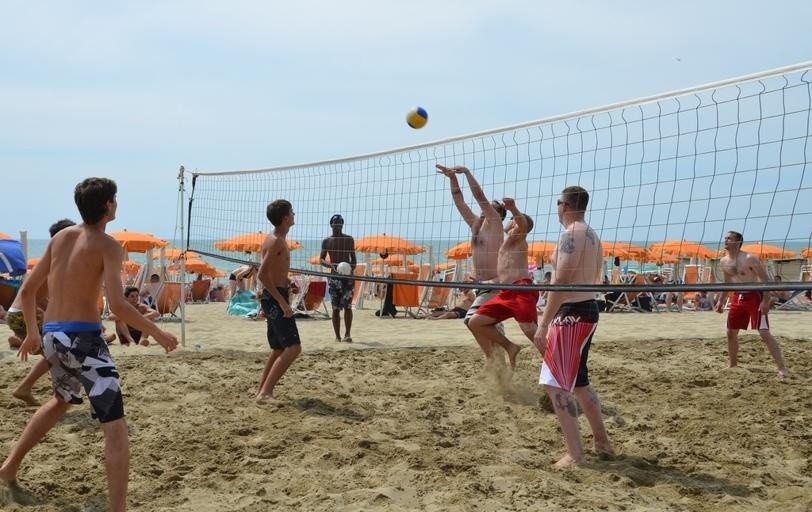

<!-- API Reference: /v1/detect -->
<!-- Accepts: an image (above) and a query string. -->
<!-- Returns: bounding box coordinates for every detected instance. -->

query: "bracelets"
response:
[449,188,460,195]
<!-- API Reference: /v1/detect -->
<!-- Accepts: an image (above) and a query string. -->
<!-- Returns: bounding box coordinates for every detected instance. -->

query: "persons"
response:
[255,199,302,405]
[603,262,723,312]
[713,231,789,376]
[101,327,116,343]
[434,164,507,371]
[536,272,551,300]
[0,176,180,510]
[468,198,547,370]
[534,186,616,468]
[8,332,23,348]
[108,287,160,345]
[6,219,79,406]
[320,213,357,341]
[770,275,812,309]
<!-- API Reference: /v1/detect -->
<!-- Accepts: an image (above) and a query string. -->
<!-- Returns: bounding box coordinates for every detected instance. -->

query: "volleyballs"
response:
[337,263,352,276]
[407,108,428,129]
